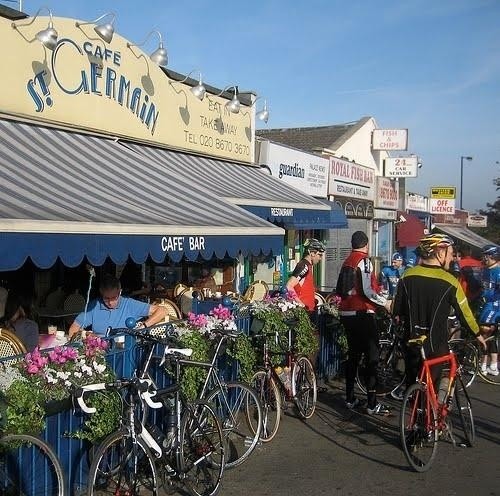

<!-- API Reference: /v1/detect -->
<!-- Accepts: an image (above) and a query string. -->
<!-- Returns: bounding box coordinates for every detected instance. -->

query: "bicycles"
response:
[244,318,317,442]
[400,325,476,473]
[77,349,225,496]
[0,434,66,496]
[473,322,500,386]
[354,319,479,397]
[87,326,263,478]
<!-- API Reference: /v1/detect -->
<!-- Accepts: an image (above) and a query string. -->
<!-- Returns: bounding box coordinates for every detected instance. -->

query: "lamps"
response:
[12,5,270,124]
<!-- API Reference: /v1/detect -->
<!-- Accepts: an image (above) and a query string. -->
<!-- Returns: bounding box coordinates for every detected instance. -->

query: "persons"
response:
[335,231,393,418]
[1,268,174,361]
[377,245,500,401]
[387,233,490,455]
[285,236,329,394]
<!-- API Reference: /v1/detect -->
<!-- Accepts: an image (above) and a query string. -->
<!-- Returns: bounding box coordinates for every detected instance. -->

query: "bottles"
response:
[274,362,288,383]
[437,374,450,405]
[165,407,177,437]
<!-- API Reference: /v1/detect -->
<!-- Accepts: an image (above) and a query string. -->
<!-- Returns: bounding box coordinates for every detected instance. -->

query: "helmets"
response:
[481,244,500,260]
[303,238,326,253]
[420,233,455,258]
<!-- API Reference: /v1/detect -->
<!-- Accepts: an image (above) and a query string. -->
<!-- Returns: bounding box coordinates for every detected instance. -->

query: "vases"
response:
[35,394,87,418]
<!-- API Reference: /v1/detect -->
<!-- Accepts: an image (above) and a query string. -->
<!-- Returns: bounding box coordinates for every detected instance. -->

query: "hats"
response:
[351,231,369,248]
[392,252,403,260]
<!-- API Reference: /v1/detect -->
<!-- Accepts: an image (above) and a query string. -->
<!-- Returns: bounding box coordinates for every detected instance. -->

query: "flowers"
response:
[166,291,320,400]
[326,295,348,354]
[0,335,121,448]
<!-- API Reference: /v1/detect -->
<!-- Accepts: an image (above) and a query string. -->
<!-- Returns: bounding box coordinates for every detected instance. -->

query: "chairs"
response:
[0,265,269,364]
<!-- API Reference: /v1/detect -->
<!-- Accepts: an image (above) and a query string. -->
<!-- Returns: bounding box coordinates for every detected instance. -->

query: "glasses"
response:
[103,290,120,301]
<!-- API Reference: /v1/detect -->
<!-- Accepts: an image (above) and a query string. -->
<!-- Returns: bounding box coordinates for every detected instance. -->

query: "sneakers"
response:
[468,367,488,375]
[487,367,499,375]
[344,398,368,408]
[367,402,392,416]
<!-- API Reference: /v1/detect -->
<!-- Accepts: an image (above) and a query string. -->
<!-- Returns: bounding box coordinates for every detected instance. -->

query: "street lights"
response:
[460,156,473,210]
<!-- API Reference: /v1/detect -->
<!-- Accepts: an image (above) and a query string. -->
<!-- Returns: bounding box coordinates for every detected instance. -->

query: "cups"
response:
[47,325,65,338]
